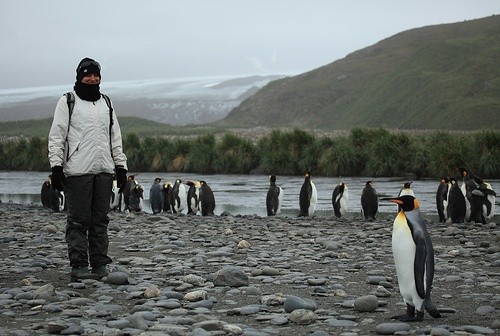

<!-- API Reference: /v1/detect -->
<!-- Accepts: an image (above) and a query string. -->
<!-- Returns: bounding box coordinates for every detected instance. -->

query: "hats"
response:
[76,58,101,83]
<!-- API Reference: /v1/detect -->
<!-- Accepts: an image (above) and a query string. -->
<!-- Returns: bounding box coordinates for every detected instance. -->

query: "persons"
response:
[47,58,127,280]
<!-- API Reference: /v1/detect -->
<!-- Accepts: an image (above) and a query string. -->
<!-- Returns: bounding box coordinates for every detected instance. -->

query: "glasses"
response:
[78,61,101,75]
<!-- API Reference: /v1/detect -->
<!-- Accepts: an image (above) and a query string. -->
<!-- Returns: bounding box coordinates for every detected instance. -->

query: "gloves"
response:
[51,166,68,191]
[115,165,128,194]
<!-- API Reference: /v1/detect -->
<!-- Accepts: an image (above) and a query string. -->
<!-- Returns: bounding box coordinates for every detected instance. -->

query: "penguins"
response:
[39,166,500,226]
[377,193,444,323]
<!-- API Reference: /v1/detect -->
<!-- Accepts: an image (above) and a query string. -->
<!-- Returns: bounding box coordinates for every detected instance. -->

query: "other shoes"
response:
[71,267,99,279]
[91,265,109,280]
